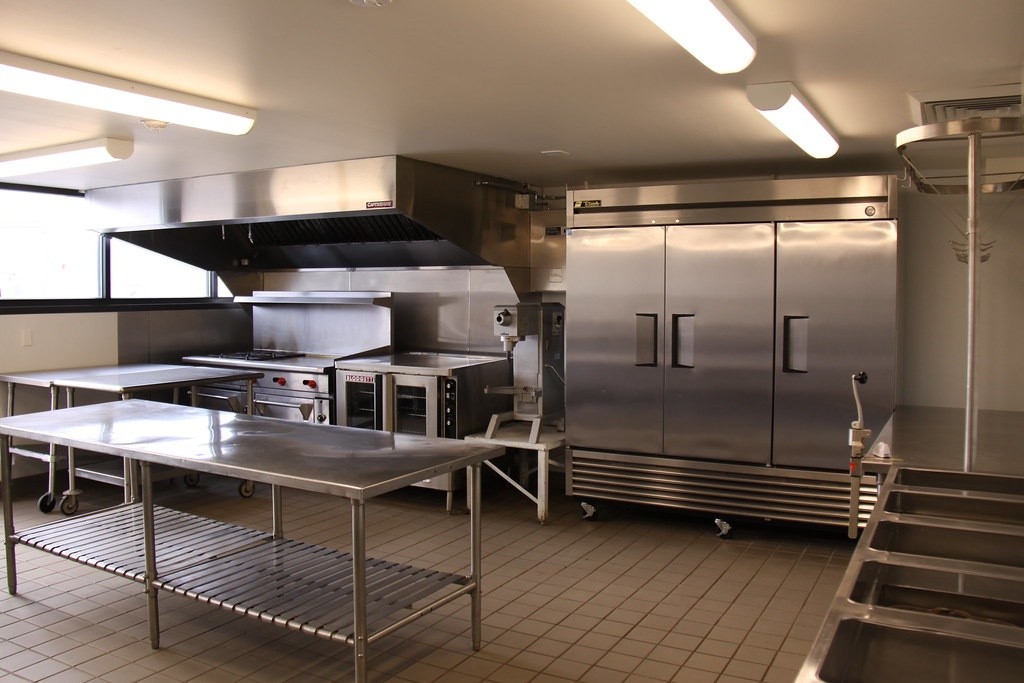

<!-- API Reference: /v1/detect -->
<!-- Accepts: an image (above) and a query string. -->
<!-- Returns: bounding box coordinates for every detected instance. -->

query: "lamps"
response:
[745,81,840,158]
[0,137,134,175]
[0,50,257,135]
[624,0,756,74]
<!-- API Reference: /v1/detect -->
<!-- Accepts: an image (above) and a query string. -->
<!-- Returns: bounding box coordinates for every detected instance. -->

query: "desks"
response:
[52,366,264,514]
[0,362,193,512]
[0,398,506,683]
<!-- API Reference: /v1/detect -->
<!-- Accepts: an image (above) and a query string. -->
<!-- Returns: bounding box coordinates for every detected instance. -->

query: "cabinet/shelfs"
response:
[563,175,907,539]
